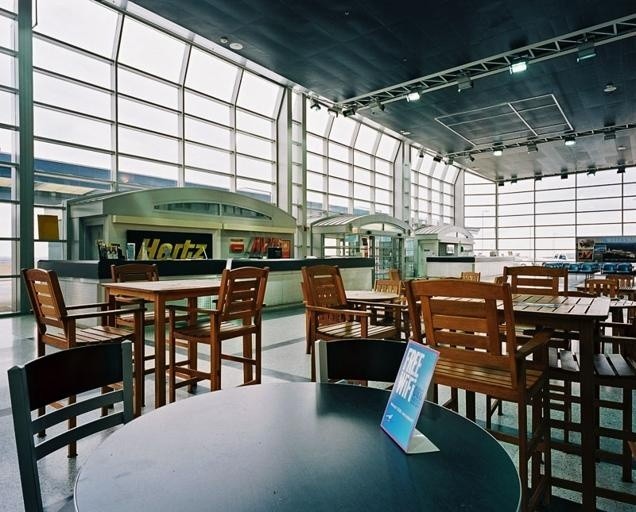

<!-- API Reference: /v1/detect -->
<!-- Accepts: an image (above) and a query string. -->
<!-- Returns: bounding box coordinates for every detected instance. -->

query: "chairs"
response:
[314,336,414,384]
[503,264,572,453]
[538,346,636,511]
[404,278,552,512]
[167,265,270,402]
[110,262,182,406]
[298,264,408,387]
[6,338,136,510]
[422,255,636,360]
[19,267,145,458]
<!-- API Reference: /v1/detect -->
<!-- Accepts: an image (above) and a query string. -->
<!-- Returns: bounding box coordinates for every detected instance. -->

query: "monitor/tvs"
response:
[267,247,283,259]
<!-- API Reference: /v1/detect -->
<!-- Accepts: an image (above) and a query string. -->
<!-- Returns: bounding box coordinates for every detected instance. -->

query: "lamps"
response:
[308,96,343,118]
[340,37,597,118]
[442,126,619,166]
[496,160,629,187]
[416,146,444,163]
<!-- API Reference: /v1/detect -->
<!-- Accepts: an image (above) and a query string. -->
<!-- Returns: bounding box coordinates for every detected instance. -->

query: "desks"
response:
[73,382,522,511]
[403,293,611,509]
[96,279,265,429]
